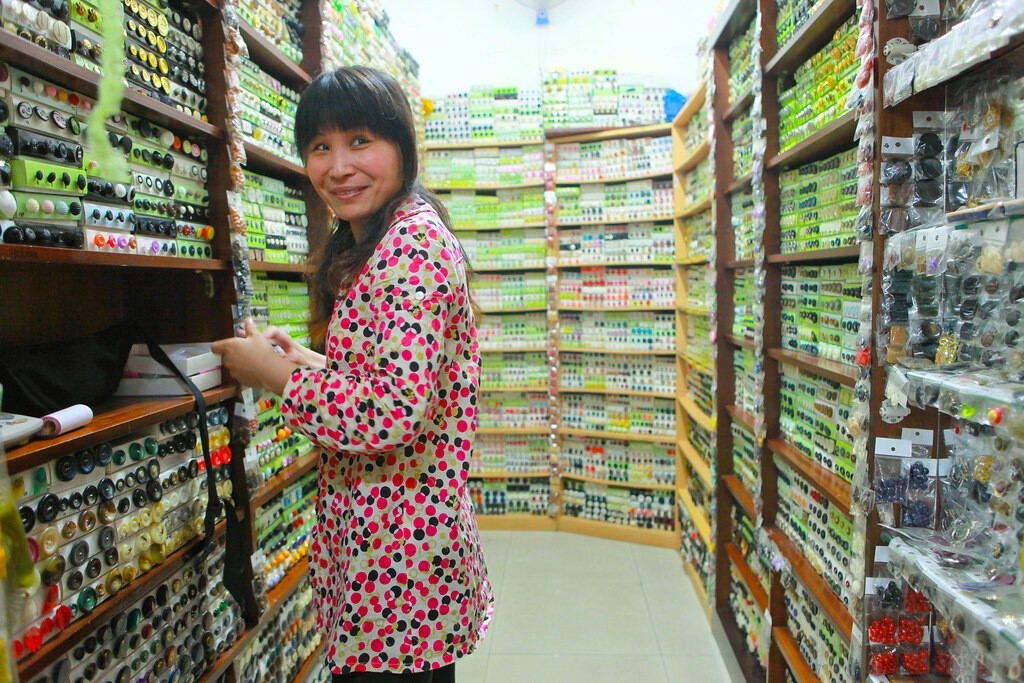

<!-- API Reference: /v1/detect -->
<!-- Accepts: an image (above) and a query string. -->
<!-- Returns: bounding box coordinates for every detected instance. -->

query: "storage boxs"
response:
[0,0,862,683]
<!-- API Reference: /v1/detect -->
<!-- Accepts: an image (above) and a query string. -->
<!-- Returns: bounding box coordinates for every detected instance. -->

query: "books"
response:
[30,404,93,437]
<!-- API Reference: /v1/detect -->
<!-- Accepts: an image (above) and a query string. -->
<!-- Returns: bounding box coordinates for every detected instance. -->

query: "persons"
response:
[211,65,495,683]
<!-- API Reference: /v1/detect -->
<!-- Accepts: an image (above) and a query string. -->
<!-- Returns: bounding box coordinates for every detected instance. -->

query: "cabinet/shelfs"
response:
[2,0,420,683]
[425,0,1024,683]
